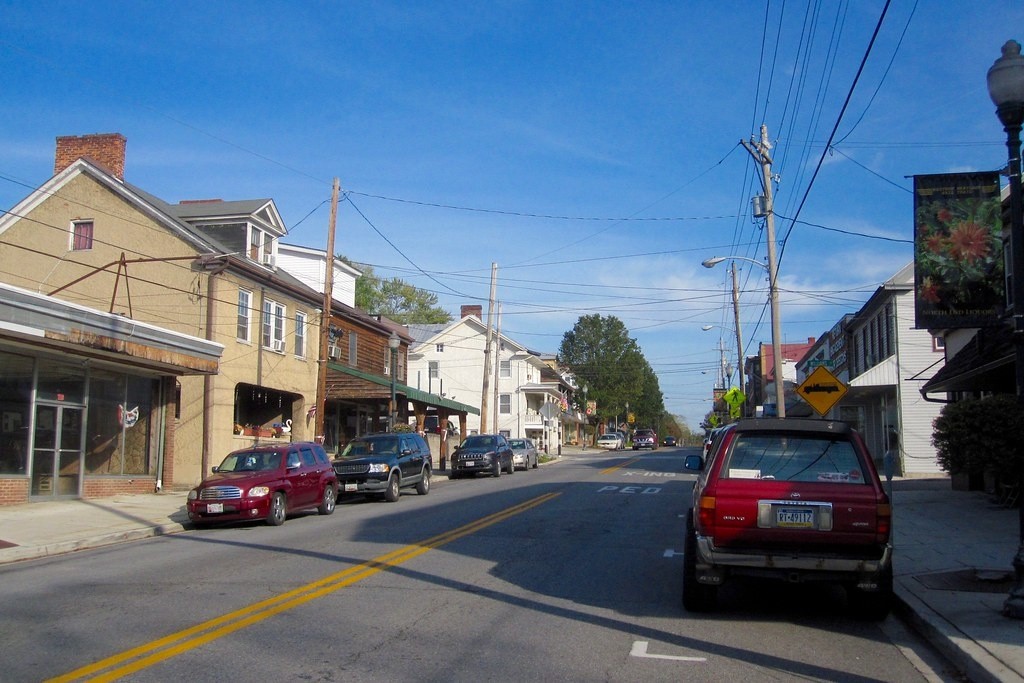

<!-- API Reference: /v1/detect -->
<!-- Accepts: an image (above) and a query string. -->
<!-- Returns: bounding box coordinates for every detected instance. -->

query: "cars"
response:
[606,432,626,450]
[502,437,539,471]
[702,423,746,467]
[597,433,622,451]
[663,435,676,447]
[683,416,896,615]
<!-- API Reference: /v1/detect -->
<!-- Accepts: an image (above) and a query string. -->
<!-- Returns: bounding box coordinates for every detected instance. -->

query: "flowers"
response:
[233,421,285,439]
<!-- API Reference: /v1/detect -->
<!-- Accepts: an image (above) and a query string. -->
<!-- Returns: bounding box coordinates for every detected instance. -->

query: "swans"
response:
[281,419,292,433]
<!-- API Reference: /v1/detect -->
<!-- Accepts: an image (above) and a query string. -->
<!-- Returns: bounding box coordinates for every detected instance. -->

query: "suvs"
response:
[449,433,514,479]
[331,431,433,500]
[187,441,336,528]
[631,428,657,450]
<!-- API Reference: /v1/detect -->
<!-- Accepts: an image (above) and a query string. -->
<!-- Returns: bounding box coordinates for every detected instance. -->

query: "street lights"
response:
[700,123,792,422]
[700,364,725,425]
[387,330,401,430]
[581,384,588,452]
[624,401,630,426]
[702,261,749,418]
